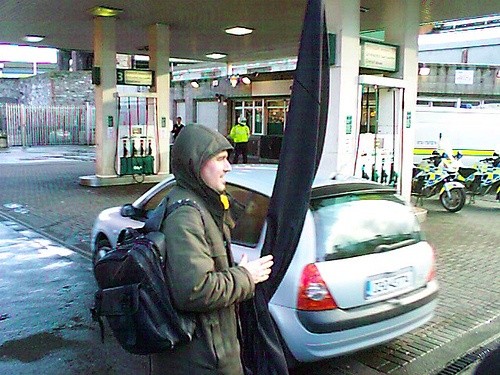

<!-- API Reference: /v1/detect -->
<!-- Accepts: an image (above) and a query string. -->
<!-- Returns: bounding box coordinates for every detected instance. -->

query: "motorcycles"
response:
[411,139,467,212]
[455,152,500,204]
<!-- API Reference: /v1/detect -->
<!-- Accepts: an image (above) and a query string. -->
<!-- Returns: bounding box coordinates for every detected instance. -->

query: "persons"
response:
[132,124,275,375]
[172,116,184,140]
[225,116,251,165]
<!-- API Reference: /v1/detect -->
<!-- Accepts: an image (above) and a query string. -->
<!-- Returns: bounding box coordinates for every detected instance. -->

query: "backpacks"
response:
[90,197,207,356]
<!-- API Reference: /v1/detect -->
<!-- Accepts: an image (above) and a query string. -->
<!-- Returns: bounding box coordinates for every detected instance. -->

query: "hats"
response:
[238,117,247,125]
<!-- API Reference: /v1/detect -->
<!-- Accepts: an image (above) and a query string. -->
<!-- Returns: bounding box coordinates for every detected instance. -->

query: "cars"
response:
[90,163,439,375]
